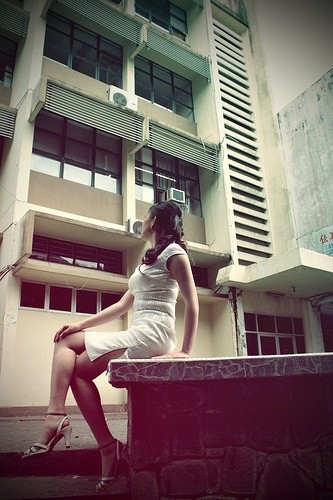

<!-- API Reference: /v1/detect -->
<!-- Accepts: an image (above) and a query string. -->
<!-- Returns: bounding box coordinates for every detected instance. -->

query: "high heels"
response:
[96,438,128,493]
[20,412,72,459]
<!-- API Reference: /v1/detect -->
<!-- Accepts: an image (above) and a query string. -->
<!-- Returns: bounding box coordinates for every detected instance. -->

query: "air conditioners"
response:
[165,187,185,204]
[125,218,143,234]
[105,85,138,111]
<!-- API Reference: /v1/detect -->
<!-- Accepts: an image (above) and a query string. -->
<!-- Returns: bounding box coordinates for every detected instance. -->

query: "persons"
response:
[21,200,200,492]
